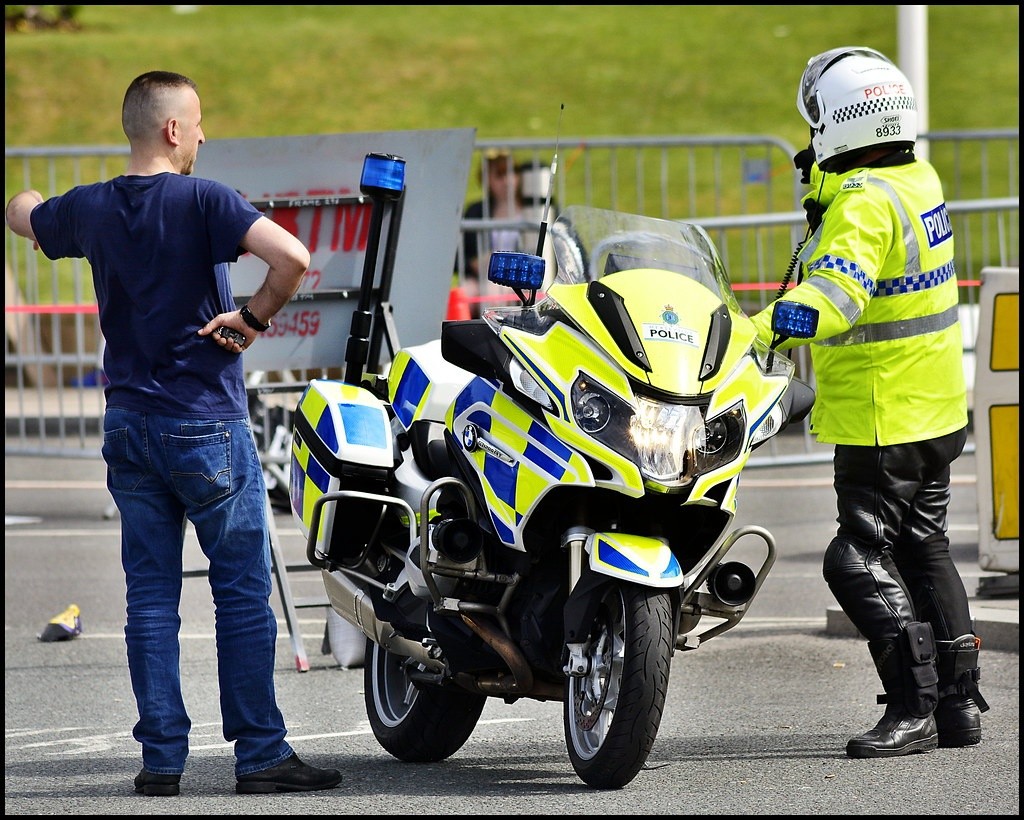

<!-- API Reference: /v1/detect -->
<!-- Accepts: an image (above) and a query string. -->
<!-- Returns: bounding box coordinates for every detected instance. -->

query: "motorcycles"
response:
[288,153,820,788]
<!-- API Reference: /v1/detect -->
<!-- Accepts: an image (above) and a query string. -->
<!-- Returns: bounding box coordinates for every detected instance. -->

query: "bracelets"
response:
[240,306,271,332]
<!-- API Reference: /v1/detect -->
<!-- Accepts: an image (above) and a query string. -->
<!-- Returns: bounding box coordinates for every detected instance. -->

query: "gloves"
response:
[794,149,816,185]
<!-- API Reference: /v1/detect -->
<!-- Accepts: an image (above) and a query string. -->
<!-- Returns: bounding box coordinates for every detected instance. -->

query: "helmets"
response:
[797,47,917,176]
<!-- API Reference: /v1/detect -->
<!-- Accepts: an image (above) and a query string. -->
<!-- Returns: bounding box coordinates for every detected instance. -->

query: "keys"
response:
[219,326,246,347]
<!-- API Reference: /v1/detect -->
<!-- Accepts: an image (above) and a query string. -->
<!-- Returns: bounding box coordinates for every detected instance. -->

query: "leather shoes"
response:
[134,767,181,797]
[933,698,981,748]
[235,751,344,795]
[846,705,938,758]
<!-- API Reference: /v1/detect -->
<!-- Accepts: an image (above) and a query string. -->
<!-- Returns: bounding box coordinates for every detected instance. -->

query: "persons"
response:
[7,70,343,795]
[747,45,989,758]
[465,148,525,277]
